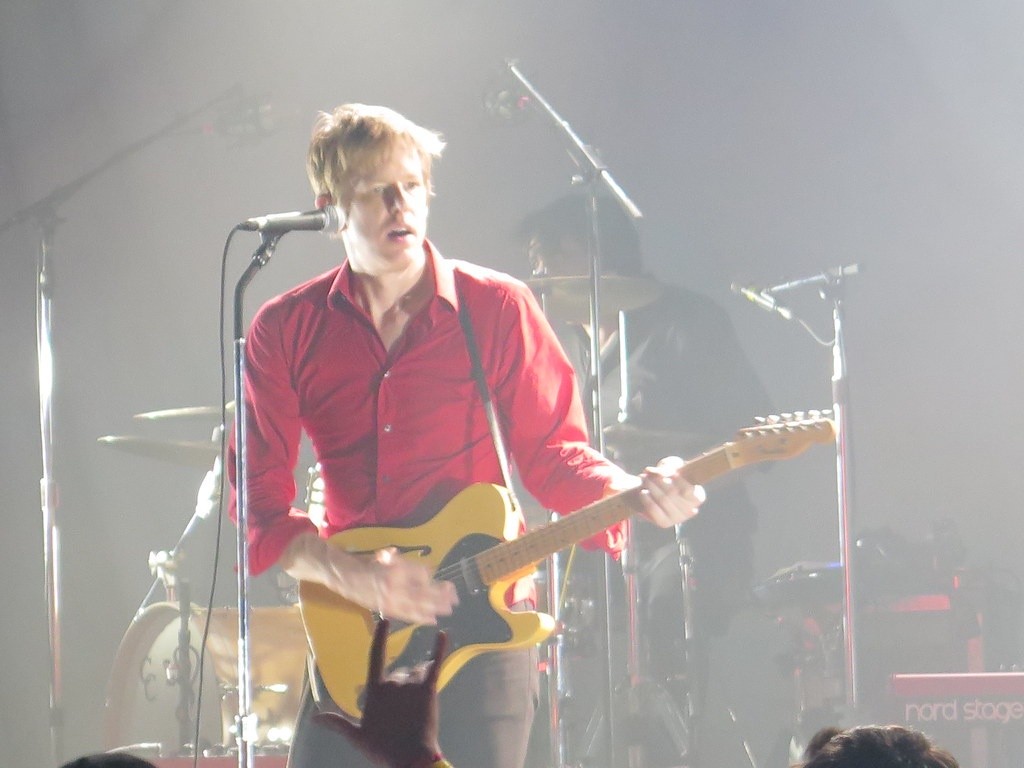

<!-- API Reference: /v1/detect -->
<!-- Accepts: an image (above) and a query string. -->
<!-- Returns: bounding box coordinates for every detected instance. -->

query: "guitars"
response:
[298,410,835,721]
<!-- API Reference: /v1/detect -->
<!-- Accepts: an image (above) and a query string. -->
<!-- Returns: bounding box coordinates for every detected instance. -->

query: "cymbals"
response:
[520,274,662,317]
[97,435,224,464]
[133,400,237,422]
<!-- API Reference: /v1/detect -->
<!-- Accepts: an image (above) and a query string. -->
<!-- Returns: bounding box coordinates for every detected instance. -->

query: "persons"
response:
[515,184,774,768]
[805,723,961,768]
[229,99,710,768]
[59,614,462,766]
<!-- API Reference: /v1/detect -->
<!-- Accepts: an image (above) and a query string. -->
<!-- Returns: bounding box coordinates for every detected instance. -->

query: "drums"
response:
[105,600,309,754]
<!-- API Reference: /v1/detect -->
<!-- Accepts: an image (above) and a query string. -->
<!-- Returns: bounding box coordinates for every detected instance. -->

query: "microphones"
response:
[481,86,538,123]
[197,401,238,520]
[238,204,347,234]
[731,281,791,321]
